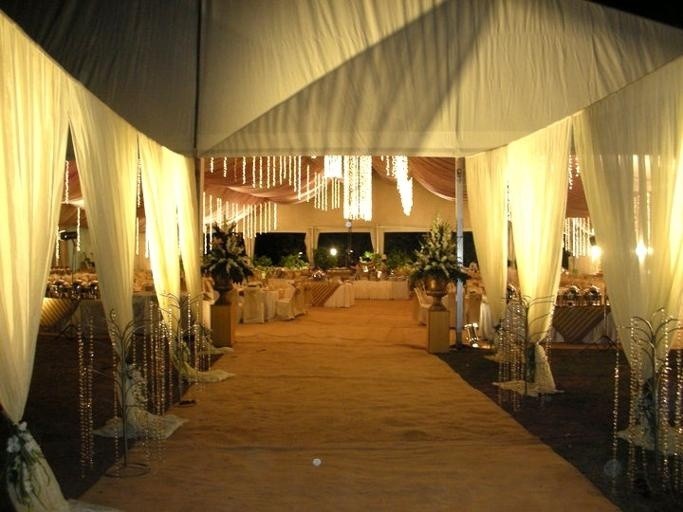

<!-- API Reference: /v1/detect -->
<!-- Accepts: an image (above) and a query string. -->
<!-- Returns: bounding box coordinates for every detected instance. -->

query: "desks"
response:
[302,280,355,308]
[238,287,294,320]
[345,277,411,300]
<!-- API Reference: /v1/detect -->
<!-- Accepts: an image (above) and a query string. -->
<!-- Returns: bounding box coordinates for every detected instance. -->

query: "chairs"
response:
[271,279,306,321]
[243,287,264,323]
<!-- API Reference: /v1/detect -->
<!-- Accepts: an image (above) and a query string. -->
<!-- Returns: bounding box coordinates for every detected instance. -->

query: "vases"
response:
[213,275,232,304]
[425,277,449,311]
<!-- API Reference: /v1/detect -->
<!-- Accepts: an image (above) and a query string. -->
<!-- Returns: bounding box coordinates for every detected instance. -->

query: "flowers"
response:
[201,223,254,283]
[411,217,471,284]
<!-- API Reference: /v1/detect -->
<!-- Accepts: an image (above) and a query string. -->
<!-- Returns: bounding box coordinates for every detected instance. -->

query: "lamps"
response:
[589,236,602,258]
